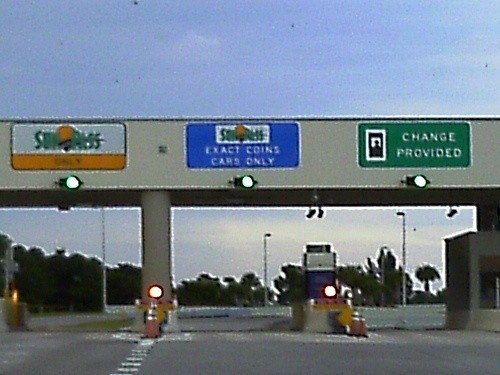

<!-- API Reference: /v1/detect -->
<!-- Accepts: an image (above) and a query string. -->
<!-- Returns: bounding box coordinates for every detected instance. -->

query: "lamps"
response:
[401,174,431,188]
[55,175,85,189]
[228,174,259,189]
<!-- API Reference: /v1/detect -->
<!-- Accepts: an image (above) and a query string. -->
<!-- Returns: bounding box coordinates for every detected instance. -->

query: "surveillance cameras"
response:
[318,210,324,218]
[307,210,316,218]
[447,209,457,217]
